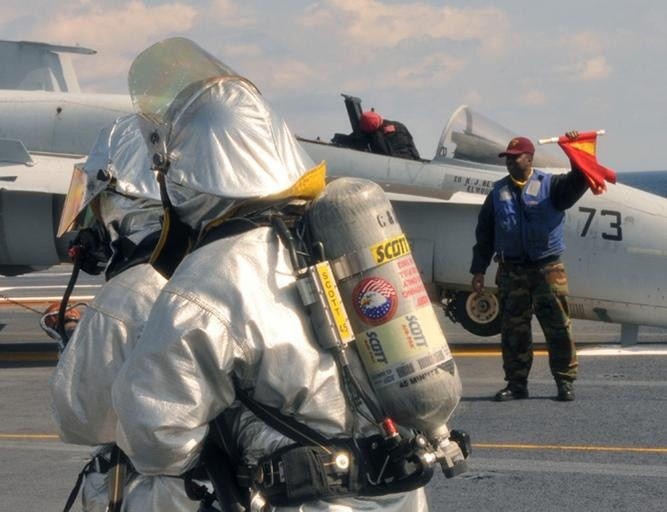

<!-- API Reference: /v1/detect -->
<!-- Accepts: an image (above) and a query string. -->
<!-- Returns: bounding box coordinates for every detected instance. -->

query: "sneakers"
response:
[557,381,576,401]
[495,383,529,401]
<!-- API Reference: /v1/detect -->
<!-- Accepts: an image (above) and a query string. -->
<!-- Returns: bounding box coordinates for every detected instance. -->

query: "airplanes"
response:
[0,41,667,338]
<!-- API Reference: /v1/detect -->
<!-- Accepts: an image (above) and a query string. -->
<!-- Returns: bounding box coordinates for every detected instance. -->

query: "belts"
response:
[497,254,561,271]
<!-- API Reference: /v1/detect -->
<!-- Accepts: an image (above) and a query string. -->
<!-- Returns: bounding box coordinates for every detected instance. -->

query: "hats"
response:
[498,136,535,157]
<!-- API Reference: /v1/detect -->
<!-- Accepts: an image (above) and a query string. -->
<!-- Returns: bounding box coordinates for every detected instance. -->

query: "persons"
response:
[108,35,431,510]
[47,113,220,510]
[467,130,591,402]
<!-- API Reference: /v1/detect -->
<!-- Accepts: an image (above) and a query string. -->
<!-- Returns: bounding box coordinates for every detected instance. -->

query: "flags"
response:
[556,131,616,196]
